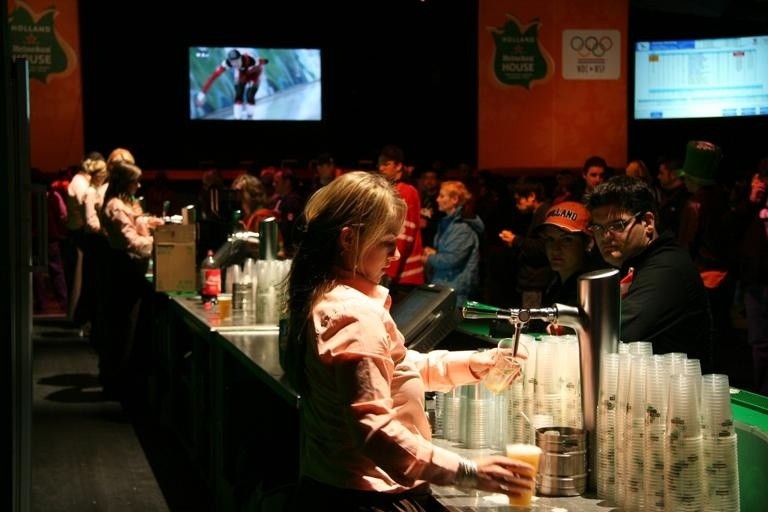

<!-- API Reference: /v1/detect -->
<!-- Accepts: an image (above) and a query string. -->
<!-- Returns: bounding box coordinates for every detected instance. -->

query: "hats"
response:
[540,201,593,235]
[228,50,241,61]
[674,139,724,186]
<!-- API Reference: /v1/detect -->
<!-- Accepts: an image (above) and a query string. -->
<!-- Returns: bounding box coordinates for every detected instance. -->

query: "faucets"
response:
[209,217,280,281]
[460,266,620,502]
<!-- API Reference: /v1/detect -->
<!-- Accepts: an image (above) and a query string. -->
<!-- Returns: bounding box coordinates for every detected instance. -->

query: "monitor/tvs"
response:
[630,35,768,121]
[186,44,326,126]
[390,281,458,349]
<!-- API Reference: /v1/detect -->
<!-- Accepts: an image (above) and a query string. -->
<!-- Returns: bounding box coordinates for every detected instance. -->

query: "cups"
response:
[216,294,234,320]
[507,444,542,507]
[233,282,253,311]
[435,348,507,450]
[498,333,583,449]
[255,258,295,322]
[596,339,743,512]
[224,257,254,293]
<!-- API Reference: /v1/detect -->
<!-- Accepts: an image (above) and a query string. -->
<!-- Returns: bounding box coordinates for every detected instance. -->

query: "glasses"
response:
[585,209,647,236]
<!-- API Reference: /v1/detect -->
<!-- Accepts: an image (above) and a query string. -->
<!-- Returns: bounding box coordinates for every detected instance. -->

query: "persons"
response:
[61,145,767,511]
[197,50,268,120]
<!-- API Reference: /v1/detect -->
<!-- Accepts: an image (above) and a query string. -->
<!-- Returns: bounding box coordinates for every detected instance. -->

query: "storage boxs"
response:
[152,224,196,292]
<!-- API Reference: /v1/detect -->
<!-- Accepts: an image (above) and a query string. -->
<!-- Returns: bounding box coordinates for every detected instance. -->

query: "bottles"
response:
[200,249,221,303]
